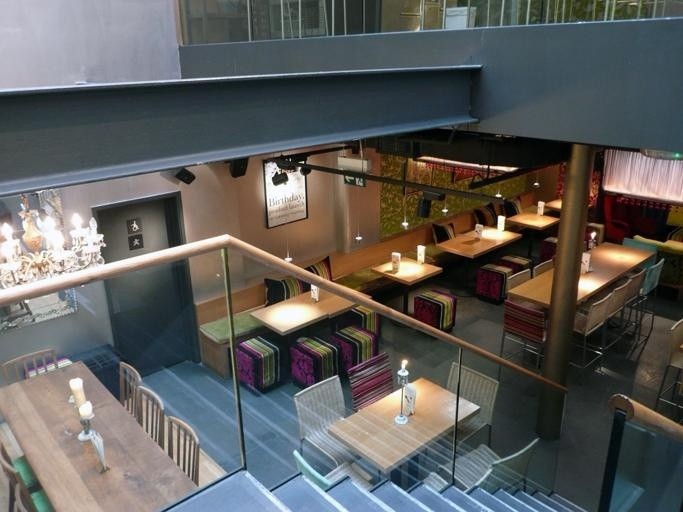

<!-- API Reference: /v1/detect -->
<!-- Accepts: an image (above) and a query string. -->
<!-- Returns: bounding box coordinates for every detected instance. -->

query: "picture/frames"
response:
[262,157,308,228]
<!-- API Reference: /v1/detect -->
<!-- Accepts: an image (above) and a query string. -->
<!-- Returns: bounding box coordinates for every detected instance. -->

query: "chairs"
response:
[505,269,531,289]
[569,292,612,387]
[0,348,200,512]
[438,438,541,494]
[497,300,549,380]
[655,319,683,411]
[417,362,499,479]
[533,259,552,278]
[294,375,361,468]
[347,351,394,413]
[602,258,665,342]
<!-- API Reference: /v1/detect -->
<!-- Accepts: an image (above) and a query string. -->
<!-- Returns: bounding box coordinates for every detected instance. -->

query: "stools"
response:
[414,290,457,331]
[329,325,379,378]
[236,335,283,393]
[502,255,533,273]
[475,262,515,303]
[292,336,340,387]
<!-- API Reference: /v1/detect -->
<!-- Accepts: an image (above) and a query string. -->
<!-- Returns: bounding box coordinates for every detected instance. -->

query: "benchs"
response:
[194,282,267,381]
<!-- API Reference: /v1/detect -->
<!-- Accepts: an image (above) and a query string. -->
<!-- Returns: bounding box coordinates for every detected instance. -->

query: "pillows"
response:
[501,197,522,218]
[265,255,333,307]
[432,223,455,245]
[472,200,496,228]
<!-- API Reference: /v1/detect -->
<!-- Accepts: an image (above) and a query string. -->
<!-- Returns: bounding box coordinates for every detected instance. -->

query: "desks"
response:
[546,197,591,213]
[505,210,561,258]
[435,225,522,285]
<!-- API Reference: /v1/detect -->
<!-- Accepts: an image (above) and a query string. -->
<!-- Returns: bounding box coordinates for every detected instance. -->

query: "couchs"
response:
[597,190,683,290]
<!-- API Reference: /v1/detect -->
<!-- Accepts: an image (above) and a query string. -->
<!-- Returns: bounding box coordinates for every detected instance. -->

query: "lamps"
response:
[0,193,105,291]
[175,127,469,220]
